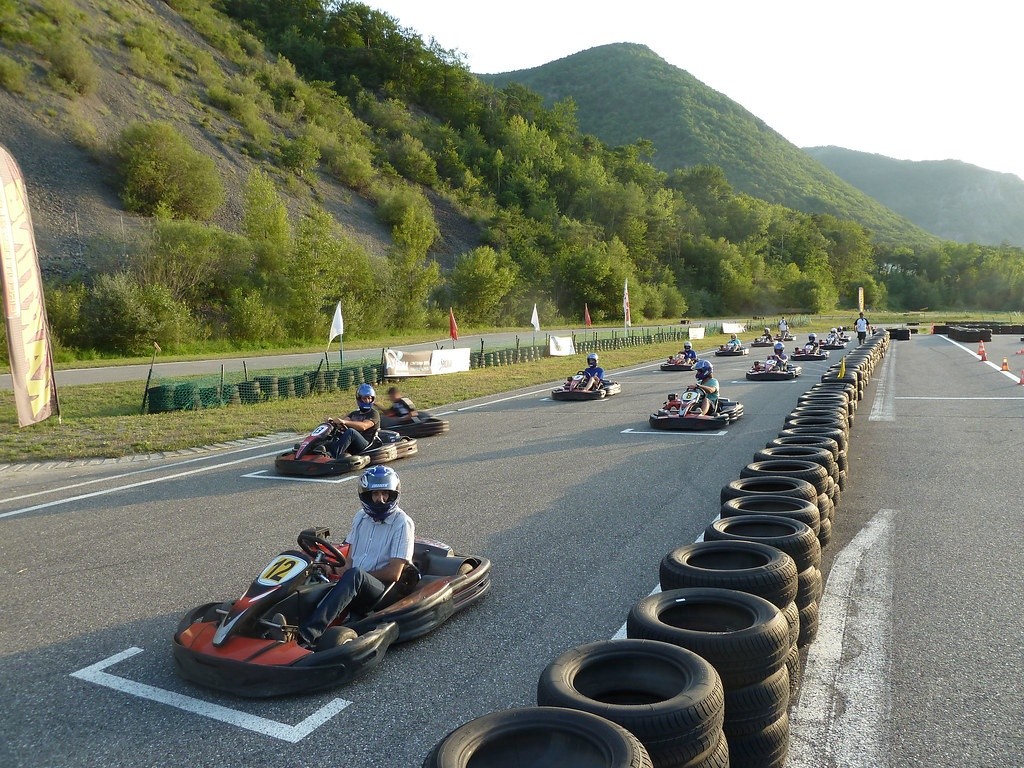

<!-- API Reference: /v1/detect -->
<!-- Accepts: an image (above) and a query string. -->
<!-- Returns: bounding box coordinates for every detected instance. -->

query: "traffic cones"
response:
[977,340,984,355]
[980,350,989,361]
[1000,356,1010,371]
[1017,370,1024,385]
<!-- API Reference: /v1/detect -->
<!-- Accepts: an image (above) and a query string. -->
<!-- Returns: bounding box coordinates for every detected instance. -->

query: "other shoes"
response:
[312,449,329,457]
[696,408,703,415]
[269,612,295,644]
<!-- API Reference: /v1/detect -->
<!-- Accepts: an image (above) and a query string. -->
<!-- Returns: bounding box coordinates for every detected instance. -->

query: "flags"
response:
[0,143,58,428]
[623,283,632,326]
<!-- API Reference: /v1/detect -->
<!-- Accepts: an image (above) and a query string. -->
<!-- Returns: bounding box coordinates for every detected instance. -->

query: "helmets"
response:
[764,327,770,333]
[691,361,713,381]
[356,384,376,410]
[358,465,401,521]
[831,327,837,334]
[731,333,737,340]
[684,341,692,351]
[808,333,817,342]
[837,326,844,332]
[587,353,598,367]
[774,342,785,354]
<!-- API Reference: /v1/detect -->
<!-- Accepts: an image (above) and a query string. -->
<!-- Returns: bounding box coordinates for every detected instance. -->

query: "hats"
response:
[859,312,864,315]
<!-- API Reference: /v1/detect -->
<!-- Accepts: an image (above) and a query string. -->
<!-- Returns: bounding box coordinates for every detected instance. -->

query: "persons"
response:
[669,342,696,365]
[778,316,788,341]
[721,333,741,351]
[853,312,871,345]
[762,327,773,341]
[768,342,788,371]
[565,353,604,391]
[837,326,845,337]
[805,333,818,355]
[658,359,718,416]
[374,386,418,422]
[830,328,839,344]
[294,384,380,459]
[220,466,415,647]
